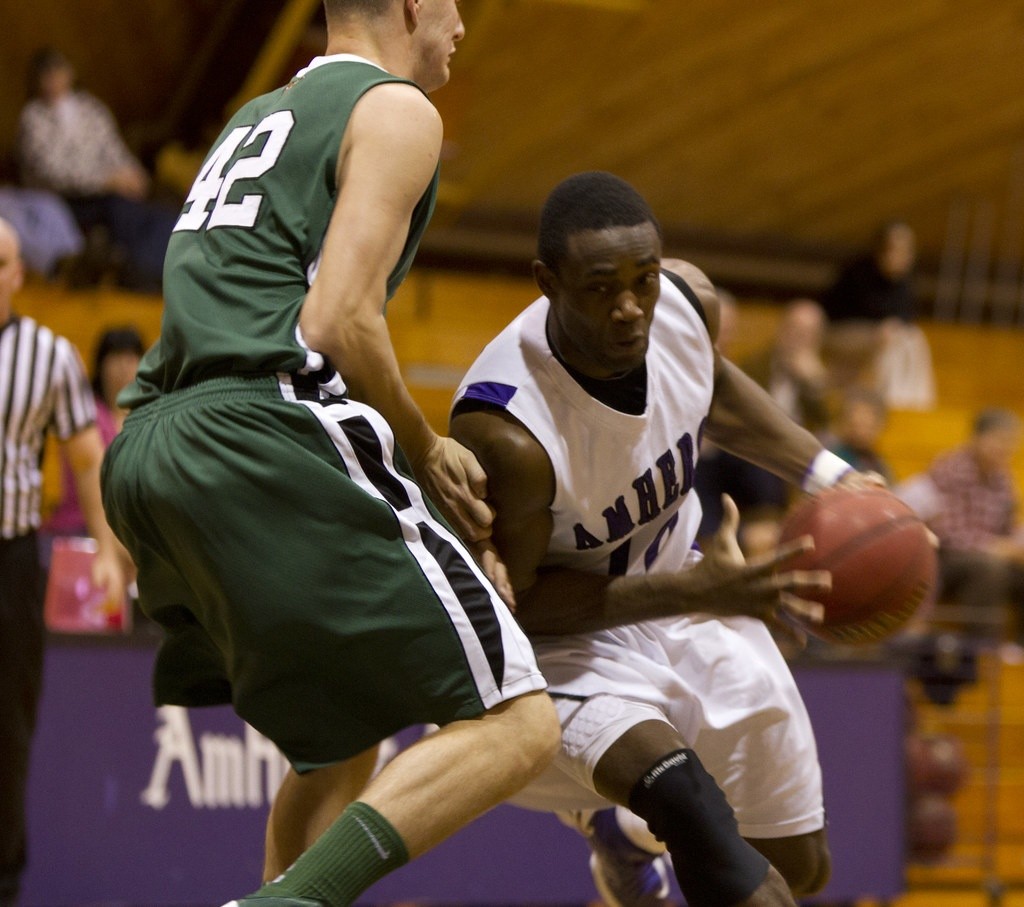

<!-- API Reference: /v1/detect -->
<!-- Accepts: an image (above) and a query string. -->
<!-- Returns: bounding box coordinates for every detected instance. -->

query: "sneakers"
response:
[551,806,669,907]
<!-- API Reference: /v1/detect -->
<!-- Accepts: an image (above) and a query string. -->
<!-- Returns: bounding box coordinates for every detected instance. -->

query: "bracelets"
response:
[803,449,848,499]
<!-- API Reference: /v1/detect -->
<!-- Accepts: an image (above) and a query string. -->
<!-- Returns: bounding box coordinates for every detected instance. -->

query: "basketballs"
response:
[778,489,935,647]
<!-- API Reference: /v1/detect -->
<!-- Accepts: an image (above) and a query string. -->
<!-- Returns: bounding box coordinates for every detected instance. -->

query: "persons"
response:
[447,171,941,907]
[714,214,1023,862]
[0,41,146,907]
[98,0,563,907]
